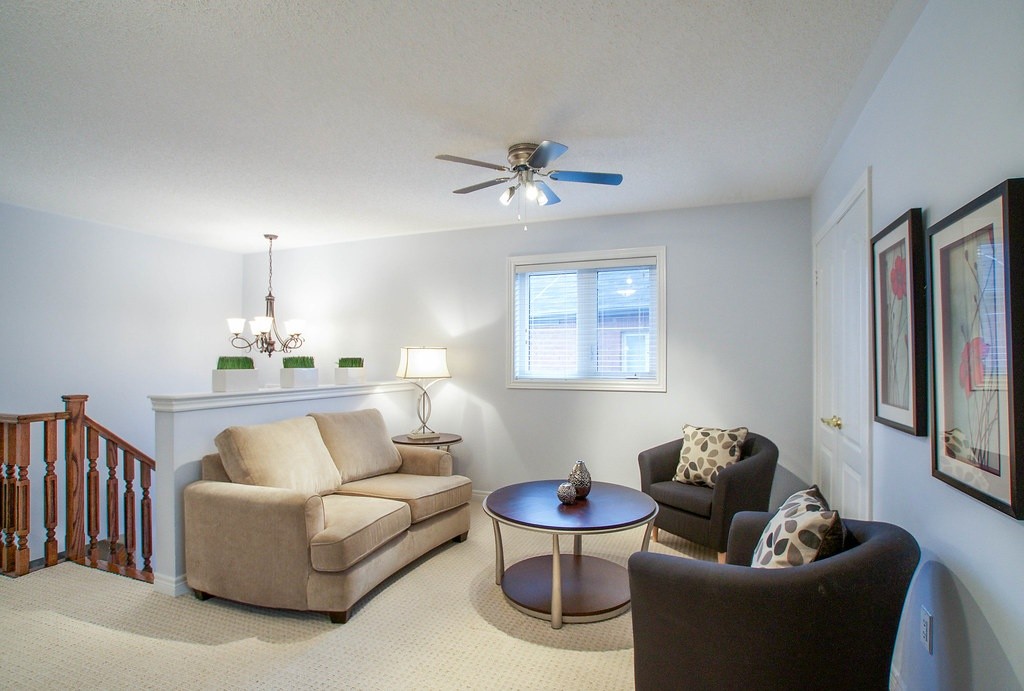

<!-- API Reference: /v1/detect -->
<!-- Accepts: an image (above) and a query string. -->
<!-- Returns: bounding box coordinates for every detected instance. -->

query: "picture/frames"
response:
[870,208,927,438]
[924,178,1023,521]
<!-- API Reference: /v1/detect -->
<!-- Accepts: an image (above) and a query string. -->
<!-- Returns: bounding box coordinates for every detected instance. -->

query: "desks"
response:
[390,433,462,453]
[481,480,659,630]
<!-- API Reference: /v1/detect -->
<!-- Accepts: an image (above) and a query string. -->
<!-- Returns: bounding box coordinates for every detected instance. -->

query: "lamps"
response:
[498,171,548,232]
[395,345,451,440]
[226,234,304,358]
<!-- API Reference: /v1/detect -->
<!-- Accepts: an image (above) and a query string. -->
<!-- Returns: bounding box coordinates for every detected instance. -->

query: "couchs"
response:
[638,433,778,565]
[627,512,920,691]
[183,409,471,625]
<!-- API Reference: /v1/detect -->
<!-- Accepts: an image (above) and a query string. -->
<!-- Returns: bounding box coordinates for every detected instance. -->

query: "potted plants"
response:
[279,357,317,388]
[211,357,256,393]
[334,358,364,385]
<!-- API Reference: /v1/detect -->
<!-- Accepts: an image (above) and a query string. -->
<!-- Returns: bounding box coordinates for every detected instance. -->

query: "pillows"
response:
[672,424,748,490]
[750,485,846,569]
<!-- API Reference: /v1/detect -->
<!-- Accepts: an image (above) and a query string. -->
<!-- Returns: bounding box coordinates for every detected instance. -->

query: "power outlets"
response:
[920,606,932,656]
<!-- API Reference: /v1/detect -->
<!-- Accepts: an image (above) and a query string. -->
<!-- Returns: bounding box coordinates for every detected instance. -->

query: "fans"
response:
[434,140,623,206]
